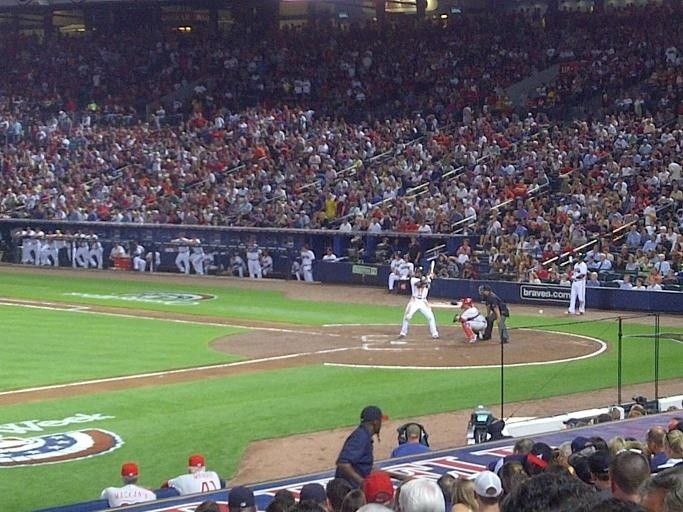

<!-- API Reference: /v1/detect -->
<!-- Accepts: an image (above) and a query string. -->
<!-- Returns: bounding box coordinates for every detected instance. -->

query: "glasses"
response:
[614,447,645,457]
[665,428,679,443]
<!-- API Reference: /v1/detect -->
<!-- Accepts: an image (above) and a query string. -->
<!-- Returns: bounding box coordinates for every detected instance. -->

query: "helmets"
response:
[478,284,491,295]
[461,298,473,309]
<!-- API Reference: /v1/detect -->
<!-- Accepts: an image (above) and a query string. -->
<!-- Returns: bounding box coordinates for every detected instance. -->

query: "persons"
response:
[361,470,397,506]
[137,19,260,231]
[608,451,652,501]
[53,230,66,268]
[273,490,294,507]
[296,245,316,282]
[499,473,601,512]
[339,489,366,512]
[102,463,157,507]
[497,462,529,486]
[19,226,36,264]
[336,407,390,488]
[609,436,626,453]
[44,230,58,266]
[228,254,246,276]
[646,427,669,469]
[647,398,683,417]
[400,268,442,342]
[300,482,328,508]
[185,232,205,275]
[391,422,431,457]
[163,453,223,496]
[192,500,222,512]
[169,233,191,274]
[658,430,683,468]
[595,496,650,512]
[267,500,290,511]
[260,21,328,241]
[567,251,589,315]
[451,478,481,512]
[110,240,126,262]
[389,252,404,295]
[639,464,683,510]
[477,1,562,285]
[458,303,488,344]
[470,469,506,512]
[562,404,647,429]
[245,233,263,280]
[551,448,575,474]
[514,438,536,455]
[290,500,327,512]
[625,437,647,454]
[330,19,390,250]
[322,247,338,263]
[396,476,446,512]
[260,251,273,276]
[442,254,476,281]
[667,417,683,431]
[476,284,510,343]
[563,3,591,251]
[533,442,556,462]
[228,485,256,512]
[0,30,136,223]
[450,5,475,254]
[129,240,147,272]
[592,0,683,288]
[399,253,413,285]
[61,227,76,267]
[71,229,89,266]
[584,450,614,490]
[29,226,46,265]
[86,230,104,270]
[437,474,456,512]
[570,437,595,458]
[357,504,394,512]
[390,16,447,252]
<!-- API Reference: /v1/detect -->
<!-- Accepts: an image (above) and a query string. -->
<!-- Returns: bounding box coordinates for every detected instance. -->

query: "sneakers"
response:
[397,333,408,339]
[480,334,491,341]
[464,336,470,344]
[500,337,509,344]
[432,334,440,340]
[469,333,477,344]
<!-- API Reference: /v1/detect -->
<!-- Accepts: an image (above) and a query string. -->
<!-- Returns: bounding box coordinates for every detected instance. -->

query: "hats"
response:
[360,406,388,423]
[561,418,579,425]
[527,443,553,467]
[588,451,613,473]
[298,482,327,504]
[120,463,138,477]
[630,396,648,403]
[570,436,596,453]
[362,470,393,505]
[567,451,595,484]
[473,469,503,499]
[226,487,256,509]
[187,455,205,467]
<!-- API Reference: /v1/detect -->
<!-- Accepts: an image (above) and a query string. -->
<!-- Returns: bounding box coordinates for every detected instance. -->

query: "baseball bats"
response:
[430,260,435,274]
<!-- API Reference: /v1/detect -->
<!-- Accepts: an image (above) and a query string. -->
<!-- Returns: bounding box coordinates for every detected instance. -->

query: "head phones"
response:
[397,423,428,440]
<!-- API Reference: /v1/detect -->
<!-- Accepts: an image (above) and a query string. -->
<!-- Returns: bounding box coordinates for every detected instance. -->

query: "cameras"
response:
[466,410,513,445]
